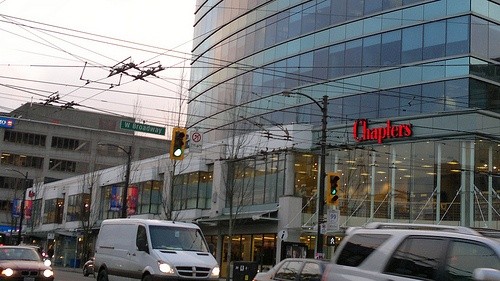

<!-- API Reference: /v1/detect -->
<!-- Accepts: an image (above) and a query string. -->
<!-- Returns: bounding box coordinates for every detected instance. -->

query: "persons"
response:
[47,245,54,261]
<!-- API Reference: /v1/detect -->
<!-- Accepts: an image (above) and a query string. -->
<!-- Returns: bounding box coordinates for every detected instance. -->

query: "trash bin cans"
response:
[70,258,79,268]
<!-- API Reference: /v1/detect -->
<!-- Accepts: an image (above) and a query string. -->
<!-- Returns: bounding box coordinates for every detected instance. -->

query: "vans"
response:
[93,219,220,280]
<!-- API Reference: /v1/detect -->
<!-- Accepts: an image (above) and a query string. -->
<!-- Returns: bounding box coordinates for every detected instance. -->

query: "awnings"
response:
[197,210,279,222]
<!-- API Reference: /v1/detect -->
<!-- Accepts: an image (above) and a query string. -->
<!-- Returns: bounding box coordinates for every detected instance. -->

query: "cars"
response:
[0,246,54,281]
[252,258,330,281]
[83,255,93,276]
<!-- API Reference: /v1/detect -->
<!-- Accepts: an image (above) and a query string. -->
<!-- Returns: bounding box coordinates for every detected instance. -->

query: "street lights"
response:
[4,168,28,245]
[281,90,329,259]
[98,143,131,218]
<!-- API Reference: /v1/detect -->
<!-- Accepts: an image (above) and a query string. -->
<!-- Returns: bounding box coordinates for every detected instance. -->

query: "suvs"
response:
[321,222,500,281]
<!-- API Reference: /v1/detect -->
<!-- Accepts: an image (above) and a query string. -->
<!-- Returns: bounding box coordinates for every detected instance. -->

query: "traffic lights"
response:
[326,235,335,246]
[327,172,342,203]
[171,127,187,159]
